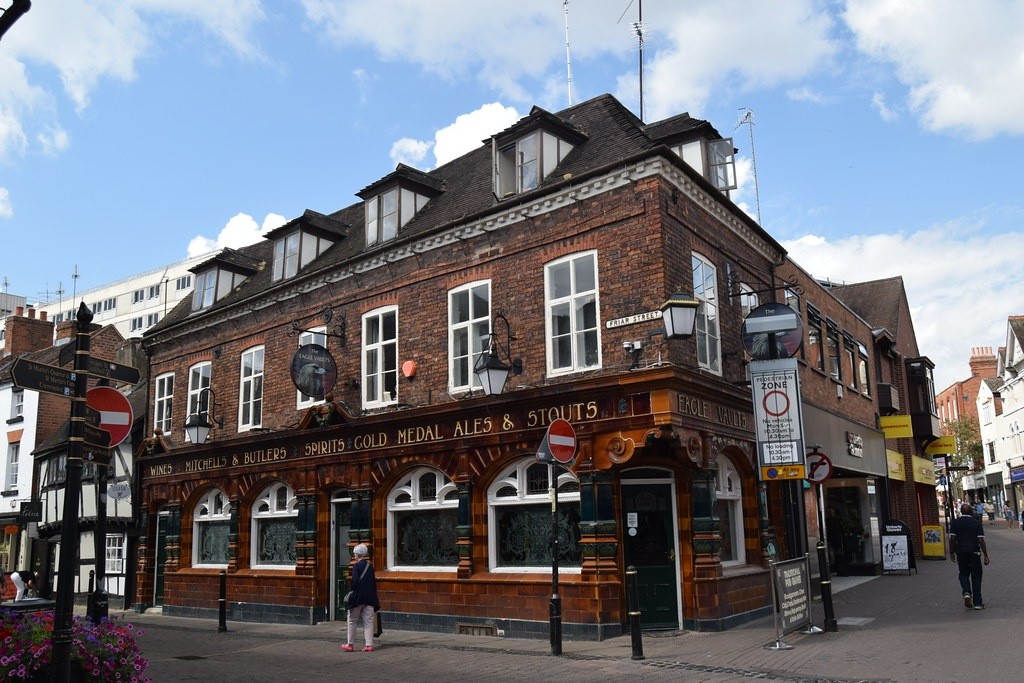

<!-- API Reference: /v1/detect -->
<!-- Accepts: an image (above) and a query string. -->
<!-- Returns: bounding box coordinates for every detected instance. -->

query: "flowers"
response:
[0,607,152,683]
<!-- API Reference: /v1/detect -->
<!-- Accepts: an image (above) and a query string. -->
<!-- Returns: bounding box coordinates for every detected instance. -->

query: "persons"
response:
[976,500,986,523]
[340,544,381,651]
[985,500,996,525]
[1004,500,1014,529]
[948,504,991,609]
[10,570,39,601]
[0,568,8,594]
[952,498,963,518]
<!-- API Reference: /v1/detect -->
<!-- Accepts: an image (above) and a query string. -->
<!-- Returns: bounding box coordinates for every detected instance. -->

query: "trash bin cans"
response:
[0,596,56,615]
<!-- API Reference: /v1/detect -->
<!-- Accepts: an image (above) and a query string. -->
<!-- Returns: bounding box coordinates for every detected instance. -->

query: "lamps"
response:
[658,285,699,340]
[183,389,223,446]
[473,317,523,397]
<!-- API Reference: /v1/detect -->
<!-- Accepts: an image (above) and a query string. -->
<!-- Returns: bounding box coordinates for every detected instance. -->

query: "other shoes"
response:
[964,595,973,608]
[974,603,985,610]
[342,643,353,652]
[361,646,373,652]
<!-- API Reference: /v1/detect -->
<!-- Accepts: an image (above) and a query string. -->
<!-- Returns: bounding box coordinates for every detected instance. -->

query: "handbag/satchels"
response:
[343,590,358,609]
[373,612,382,638]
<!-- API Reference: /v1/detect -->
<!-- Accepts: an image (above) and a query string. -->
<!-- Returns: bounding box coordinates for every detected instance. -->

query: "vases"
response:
[11,656,86,683]
